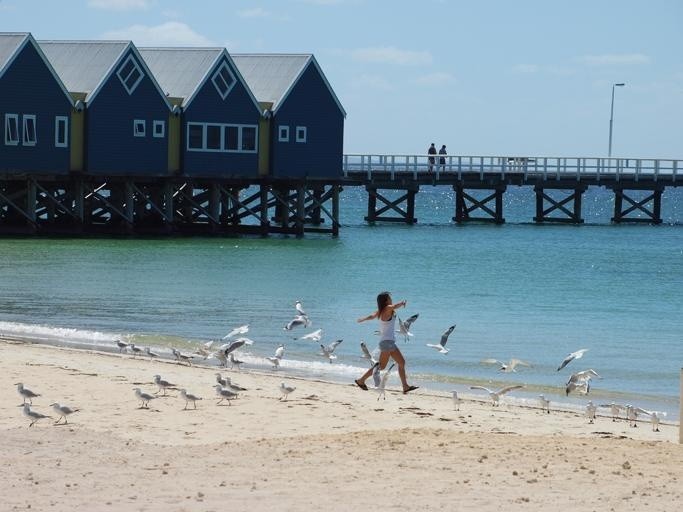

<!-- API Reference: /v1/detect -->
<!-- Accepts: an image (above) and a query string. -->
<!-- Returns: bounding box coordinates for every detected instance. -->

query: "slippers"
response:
[355,376,369,391]
[404,385,419,393]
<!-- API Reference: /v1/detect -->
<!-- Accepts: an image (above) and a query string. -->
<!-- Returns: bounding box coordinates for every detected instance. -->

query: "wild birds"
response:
[450,390,464,410]
[14,383,41,406]
[425,325,456,355]
[114,325,396,409]
[479,359,530,372]
[283,300,312,330]
[539,395,551,414]
[470,385,524,406]
[17,403,53,428]
[50,403,79,425]
[566,370,669,432]
[557,348,589,372]
[395,313,419,343]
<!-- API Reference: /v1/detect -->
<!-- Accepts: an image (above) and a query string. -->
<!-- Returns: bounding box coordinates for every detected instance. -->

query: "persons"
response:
[354,291,419,393]
[439,145,447,171]
[427,143,436,173]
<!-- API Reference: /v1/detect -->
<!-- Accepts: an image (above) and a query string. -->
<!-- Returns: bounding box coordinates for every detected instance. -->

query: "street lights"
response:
[607,83,627,171]
